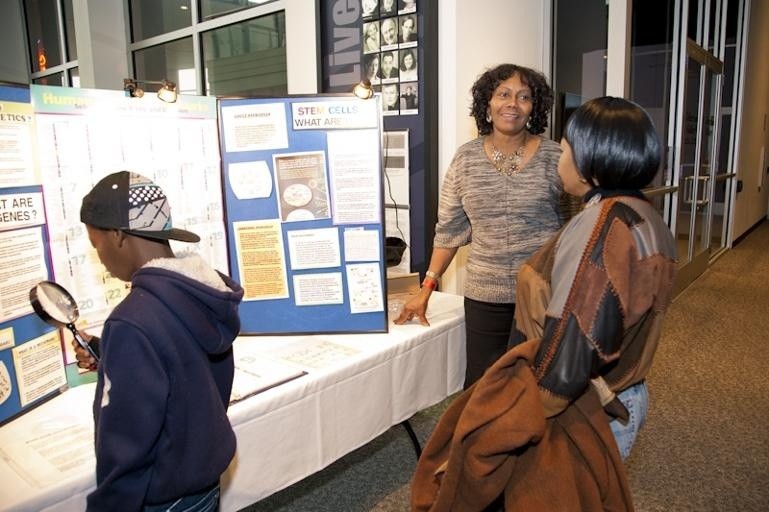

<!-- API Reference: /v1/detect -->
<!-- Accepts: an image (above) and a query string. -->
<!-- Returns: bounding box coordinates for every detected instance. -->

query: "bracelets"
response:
[425,271,441,283]
[421,278,438,291]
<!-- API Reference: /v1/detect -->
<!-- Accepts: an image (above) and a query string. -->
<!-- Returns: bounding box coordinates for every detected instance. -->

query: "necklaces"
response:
[490,127,530,177]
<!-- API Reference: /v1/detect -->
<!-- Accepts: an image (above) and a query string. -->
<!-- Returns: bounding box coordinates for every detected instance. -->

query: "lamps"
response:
[124,78,178,104]
[353,78,375,100]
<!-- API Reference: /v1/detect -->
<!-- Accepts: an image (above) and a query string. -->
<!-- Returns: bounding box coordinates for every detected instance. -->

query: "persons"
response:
[392,63,583,390]
[504,96,681,466]
[70,169,245,512]
[361,0,418,111]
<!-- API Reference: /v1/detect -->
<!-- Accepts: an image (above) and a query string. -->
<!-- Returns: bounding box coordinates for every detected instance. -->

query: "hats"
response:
[81,171,201,242]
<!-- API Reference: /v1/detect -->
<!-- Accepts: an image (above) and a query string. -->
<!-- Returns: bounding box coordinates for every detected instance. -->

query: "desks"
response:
[0,288,466,512]
